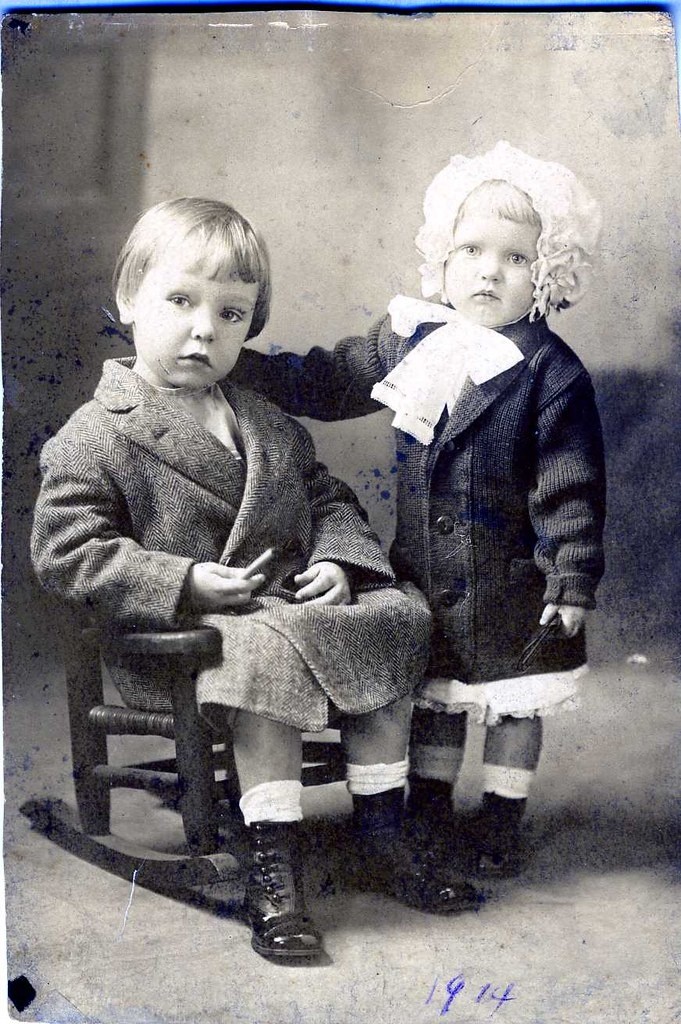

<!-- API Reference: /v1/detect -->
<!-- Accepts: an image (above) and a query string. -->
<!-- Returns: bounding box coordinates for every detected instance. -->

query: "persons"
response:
[29,196,485,959]
[226,140,608,879]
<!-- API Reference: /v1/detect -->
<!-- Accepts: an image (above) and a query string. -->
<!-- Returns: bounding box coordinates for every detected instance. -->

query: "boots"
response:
[345,786,476,913]
[239,818,325,956]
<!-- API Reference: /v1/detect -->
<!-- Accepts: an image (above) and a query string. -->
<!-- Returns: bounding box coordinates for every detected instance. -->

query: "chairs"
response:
[14,423,358,897]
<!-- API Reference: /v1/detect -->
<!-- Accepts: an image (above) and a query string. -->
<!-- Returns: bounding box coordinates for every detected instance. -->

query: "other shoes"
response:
[405,794,454,867]
[470,792,525,874]
